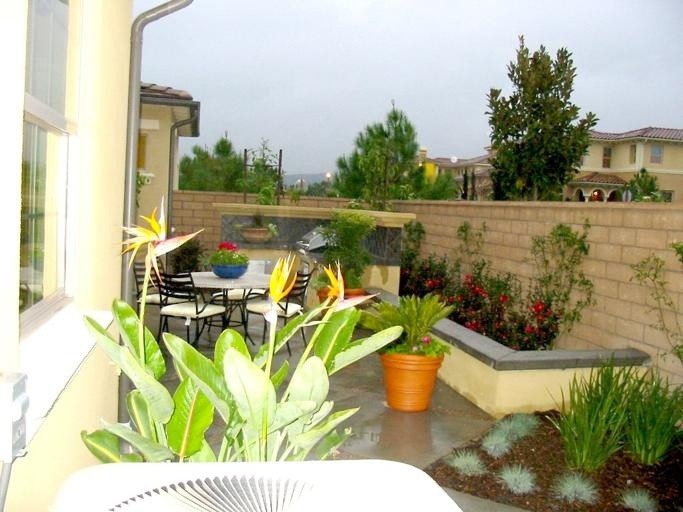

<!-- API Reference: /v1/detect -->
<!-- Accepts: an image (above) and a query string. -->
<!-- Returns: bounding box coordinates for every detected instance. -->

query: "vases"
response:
[209,262,248,278]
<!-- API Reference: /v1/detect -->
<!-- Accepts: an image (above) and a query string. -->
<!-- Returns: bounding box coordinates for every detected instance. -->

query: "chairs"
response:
[210,259,268,334]
[243,268,319,355]
[131,258,201,336]
[153,268,227,348]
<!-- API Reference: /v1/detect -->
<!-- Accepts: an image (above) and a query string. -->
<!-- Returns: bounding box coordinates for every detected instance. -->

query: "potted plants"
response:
[312,210,377,322]
[237,209,270,242]
[355,292,460,416]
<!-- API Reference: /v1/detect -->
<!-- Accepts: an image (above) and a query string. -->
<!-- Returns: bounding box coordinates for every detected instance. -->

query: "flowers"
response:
[378,334,451,358]
[206,240,251,266]
[72,193,404,462]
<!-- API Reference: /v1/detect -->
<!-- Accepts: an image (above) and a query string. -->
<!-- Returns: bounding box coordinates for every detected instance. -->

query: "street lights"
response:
[326,173,332,181]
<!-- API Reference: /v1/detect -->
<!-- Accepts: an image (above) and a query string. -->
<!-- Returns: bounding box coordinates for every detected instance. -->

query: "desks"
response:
[170,269,279,341]
[49,460,467,512]
